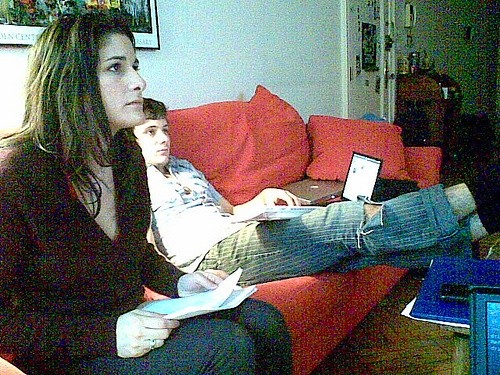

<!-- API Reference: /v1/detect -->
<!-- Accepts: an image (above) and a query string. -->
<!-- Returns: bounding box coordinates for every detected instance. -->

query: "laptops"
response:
[282,178,345,203]
[324,151,384,207]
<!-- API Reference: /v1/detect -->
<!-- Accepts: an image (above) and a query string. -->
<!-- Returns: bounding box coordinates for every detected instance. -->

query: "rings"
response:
[150,339,156,349]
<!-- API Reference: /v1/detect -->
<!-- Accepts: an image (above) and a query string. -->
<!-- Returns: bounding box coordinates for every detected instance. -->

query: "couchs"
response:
[140,83,442,374]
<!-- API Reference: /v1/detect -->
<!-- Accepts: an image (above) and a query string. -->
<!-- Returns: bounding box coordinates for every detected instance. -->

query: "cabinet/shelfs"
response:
[397,72,464,153]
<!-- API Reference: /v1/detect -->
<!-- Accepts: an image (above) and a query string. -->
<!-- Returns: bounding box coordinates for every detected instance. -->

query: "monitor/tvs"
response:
[470,286,500,375]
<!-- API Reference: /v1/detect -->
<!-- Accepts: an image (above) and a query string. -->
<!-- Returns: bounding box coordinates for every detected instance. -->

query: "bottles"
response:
[409,52,420,76]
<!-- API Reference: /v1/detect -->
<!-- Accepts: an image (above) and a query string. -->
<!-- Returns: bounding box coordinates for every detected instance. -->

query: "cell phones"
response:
[438,282,473,303]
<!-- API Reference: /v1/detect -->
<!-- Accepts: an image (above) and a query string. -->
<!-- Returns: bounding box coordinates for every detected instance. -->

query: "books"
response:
[140,268,258,320]
[230,206,321,224]
[410,256,500,325]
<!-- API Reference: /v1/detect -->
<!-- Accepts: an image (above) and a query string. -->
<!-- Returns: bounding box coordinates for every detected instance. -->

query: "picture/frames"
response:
[1,0,161,50]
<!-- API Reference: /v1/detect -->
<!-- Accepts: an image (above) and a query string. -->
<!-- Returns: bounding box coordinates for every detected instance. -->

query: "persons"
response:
[0,14,295,375]
[134,99,500,291]
